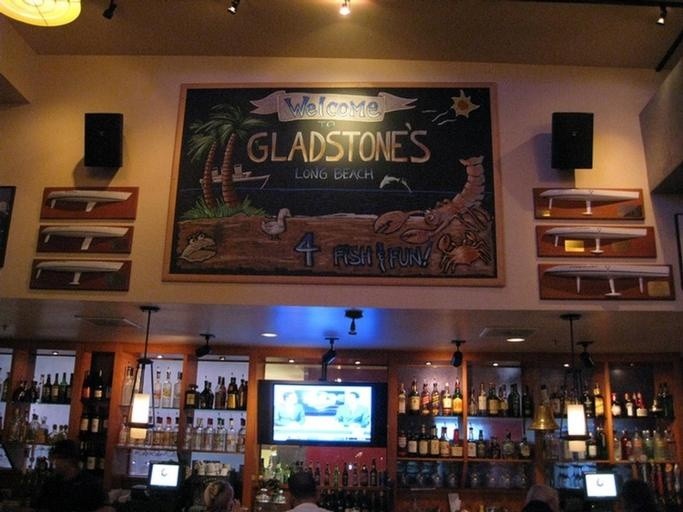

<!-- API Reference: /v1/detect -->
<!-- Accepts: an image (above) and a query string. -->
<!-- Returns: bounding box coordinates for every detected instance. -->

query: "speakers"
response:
[550,112,594,170]
[83,112,124,169]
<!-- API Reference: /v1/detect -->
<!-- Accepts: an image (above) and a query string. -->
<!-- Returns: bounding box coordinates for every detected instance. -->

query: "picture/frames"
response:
[159,78,508,290]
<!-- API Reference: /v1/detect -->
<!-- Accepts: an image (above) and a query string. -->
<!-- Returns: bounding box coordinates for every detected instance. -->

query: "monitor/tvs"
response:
[581,470,619,499]
[147,459,183,489]
[267,380,372,442]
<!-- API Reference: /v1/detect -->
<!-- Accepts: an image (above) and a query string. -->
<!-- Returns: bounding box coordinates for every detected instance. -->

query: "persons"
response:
[196,479,245,512]
[272,388,307,432]
[29,440,110,512]
[333,390,369,432]
[284,471,332,511]
[616,478,663,511]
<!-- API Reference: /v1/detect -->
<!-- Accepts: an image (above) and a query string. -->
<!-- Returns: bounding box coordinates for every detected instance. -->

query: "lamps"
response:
[558,312,592,444]
[227,1,241,15]
[656,6,668,24]
[449,339,467,367]
[323,336,340,364]
[195,331,215,358]
[123,306,164,433]
[575,338,597,369]
[99,0,119,20]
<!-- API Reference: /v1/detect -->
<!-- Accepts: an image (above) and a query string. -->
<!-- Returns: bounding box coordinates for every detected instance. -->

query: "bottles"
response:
[0,354,248,477]
[260,452,395,512]
[11,477,44,507]
[399,378,683,491]
